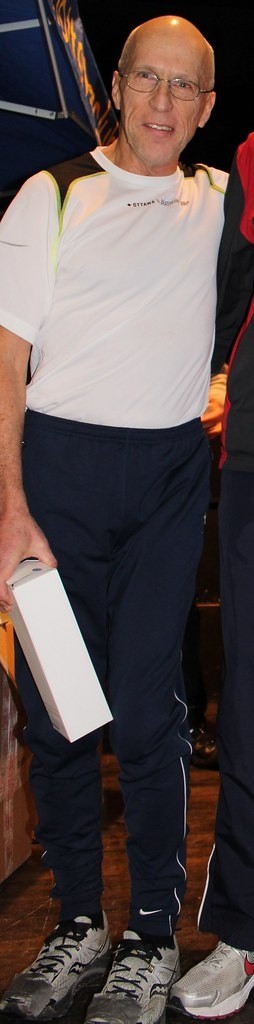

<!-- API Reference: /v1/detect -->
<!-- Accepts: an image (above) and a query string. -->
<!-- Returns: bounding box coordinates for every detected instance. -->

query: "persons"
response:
[165,131,254,1019]
[0,16,230,1024]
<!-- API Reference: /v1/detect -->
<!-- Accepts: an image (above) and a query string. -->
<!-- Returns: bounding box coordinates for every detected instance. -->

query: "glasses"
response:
[118,69,216,99]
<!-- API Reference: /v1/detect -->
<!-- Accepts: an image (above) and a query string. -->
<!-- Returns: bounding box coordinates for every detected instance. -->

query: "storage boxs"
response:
[4,558,113,743]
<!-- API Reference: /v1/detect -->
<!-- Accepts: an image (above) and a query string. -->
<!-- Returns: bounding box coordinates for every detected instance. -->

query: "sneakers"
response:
[172,943,254,1023]
[85,930,182,1024]
[0,909,110,1024]
